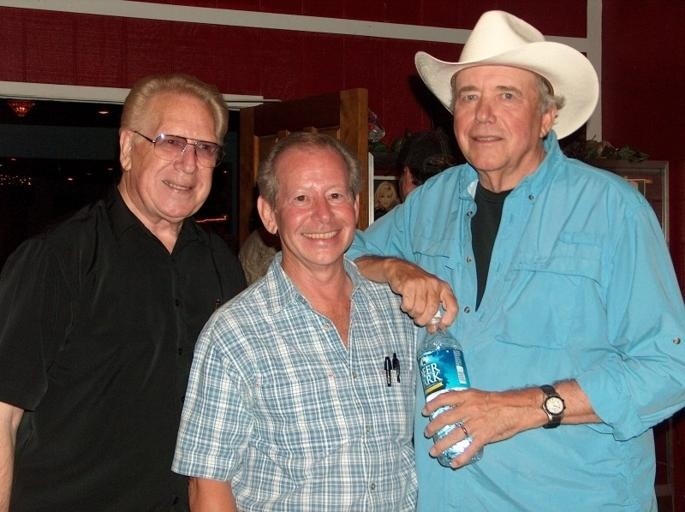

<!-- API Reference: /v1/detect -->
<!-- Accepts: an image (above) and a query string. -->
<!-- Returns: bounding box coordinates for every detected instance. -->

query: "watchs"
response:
[539,385,565,430]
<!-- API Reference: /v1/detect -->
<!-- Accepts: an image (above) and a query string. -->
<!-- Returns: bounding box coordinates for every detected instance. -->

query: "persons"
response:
[402,130,455,191]
[170,130,419,512]
[374,181,400,221]
[0,69,247,511]
[342,10,684,512]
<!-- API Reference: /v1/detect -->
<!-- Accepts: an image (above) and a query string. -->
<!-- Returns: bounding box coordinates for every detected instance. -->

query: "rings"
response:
[460,424,468,438]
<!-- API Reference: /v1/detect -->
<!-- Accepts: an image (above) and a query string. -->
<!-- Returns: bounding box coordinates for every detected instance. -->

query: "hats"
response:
[414,9,600,141]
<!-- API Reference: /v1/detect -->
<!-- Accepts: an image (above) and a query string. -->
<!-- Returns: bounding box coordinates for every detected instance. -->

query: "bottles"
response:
[417,311,484,467]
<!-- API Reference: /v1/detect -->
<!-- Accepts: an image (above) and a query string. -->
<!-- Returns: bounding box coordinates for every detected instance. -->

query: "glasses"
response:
[132,130,223,168]
